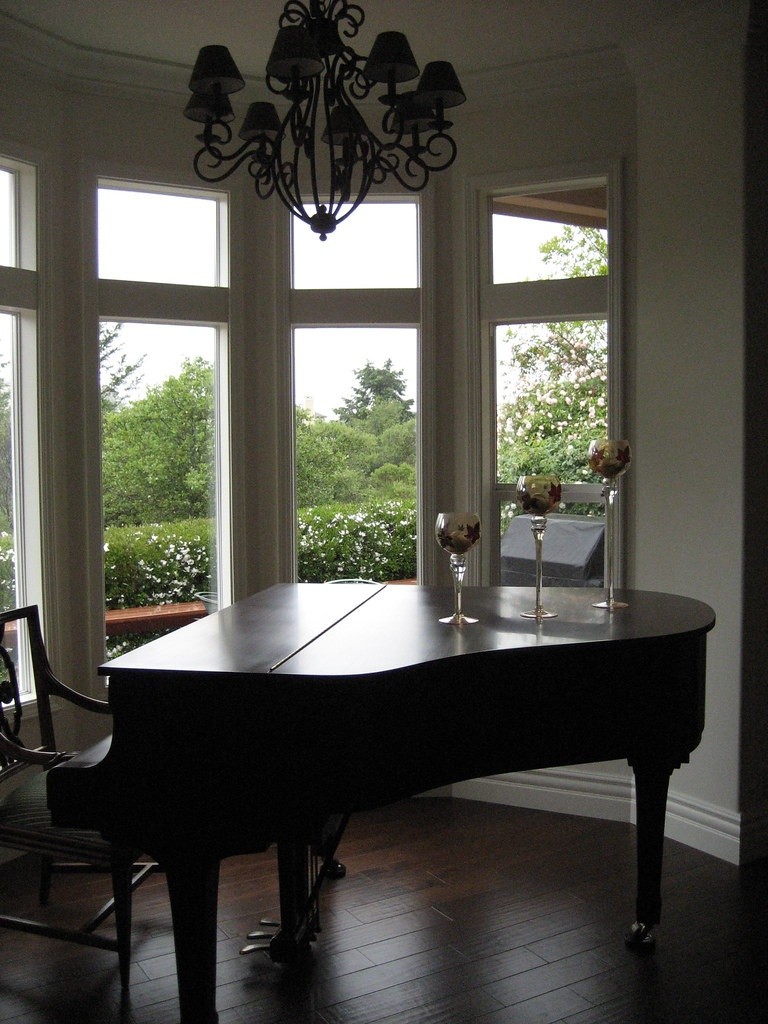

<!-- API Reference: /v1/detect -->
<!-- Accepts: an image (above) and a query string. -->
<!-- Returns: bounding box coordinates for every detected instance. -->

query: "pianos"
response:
[44,580,718,1024]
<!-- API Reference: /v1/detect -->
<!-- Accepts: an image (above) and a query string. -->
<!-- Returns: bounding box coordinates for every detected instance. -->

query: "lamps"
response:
[183,0,467,241]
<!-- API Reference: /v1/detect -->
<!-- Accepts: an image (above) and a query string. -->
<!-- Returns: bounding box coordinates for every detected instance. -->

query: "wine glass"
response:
[588,438,632,609]
[435,512,481,624]
[515,475,562,617]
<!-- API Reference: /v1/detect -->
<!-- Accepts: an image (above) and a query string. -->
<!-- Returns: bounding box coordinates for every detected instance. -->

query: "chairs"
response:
[0,604,166,989]
